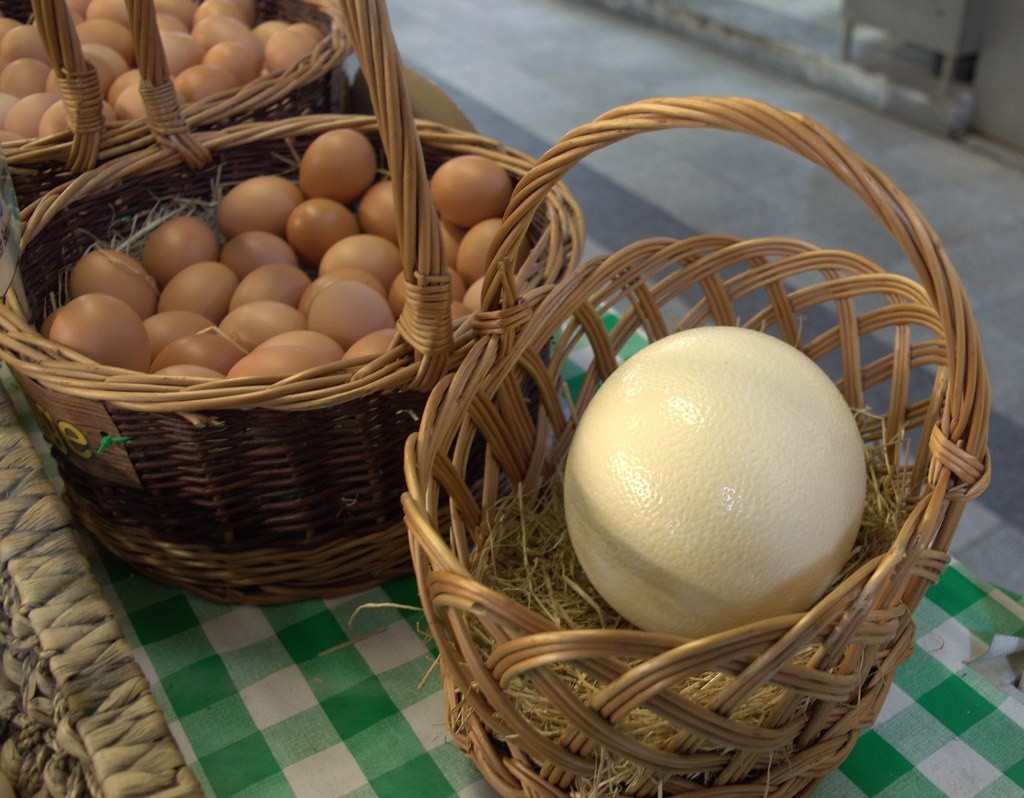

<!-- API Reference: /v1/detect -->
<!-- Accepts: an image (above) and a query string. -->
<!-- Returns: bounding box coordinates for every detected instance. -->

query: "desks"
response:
[0,144,1024,796]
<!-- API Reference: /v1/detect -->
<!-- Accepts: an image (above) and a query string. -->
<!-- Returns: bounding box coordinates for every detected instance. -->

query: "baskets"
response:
[0,1,992,798]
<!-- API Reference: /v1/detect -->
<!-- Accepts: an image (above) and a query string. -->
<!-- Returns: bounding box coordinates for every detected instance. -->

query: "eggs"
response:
[41,126,526,378]
[0,0,326,145]
